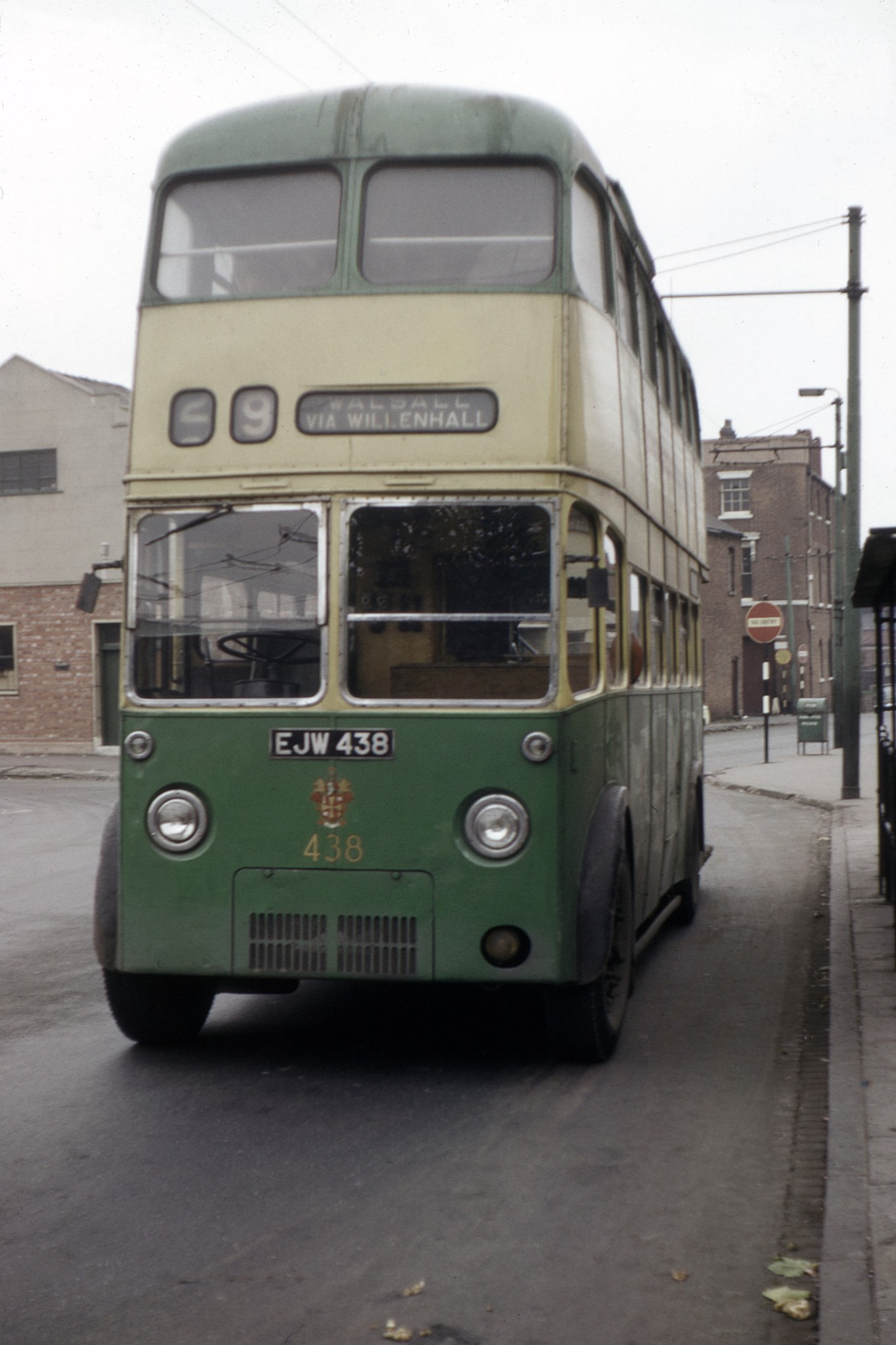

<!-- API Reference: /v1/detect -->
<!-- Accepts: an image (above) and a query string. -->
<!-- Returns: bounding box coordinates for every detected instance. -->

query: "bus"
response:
[76,79,716,1068]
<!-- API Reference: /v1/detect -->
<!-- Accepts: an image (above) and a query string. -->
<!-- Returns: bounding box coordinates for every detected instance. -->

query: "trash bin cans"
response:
[796,697,827,743]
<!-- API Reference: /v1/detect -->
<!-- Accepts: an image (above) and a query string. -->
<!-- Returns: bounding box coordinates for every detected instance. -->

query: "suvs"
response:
[872,683,893,713]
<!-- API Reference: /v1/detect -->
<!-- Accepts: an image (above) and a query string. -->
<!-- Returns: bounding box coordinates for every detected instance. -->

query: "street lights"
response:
[798,384,845,747]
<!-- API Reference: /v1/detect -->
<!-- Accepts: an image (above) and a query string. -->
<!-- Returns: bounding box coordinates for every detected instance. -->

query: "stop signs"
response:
[796,644,809,663]
[745,601,783,644]
